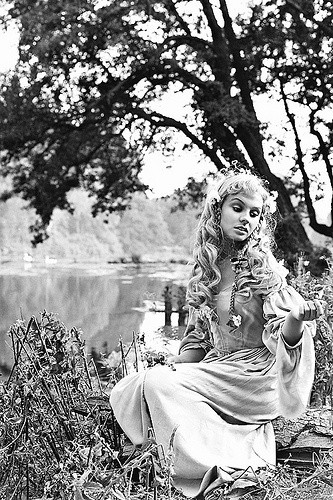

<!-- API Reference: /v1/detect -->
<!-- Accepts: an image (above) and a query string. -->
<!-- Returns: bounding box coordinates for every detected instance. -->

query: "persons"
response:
[109,168,324,498]
